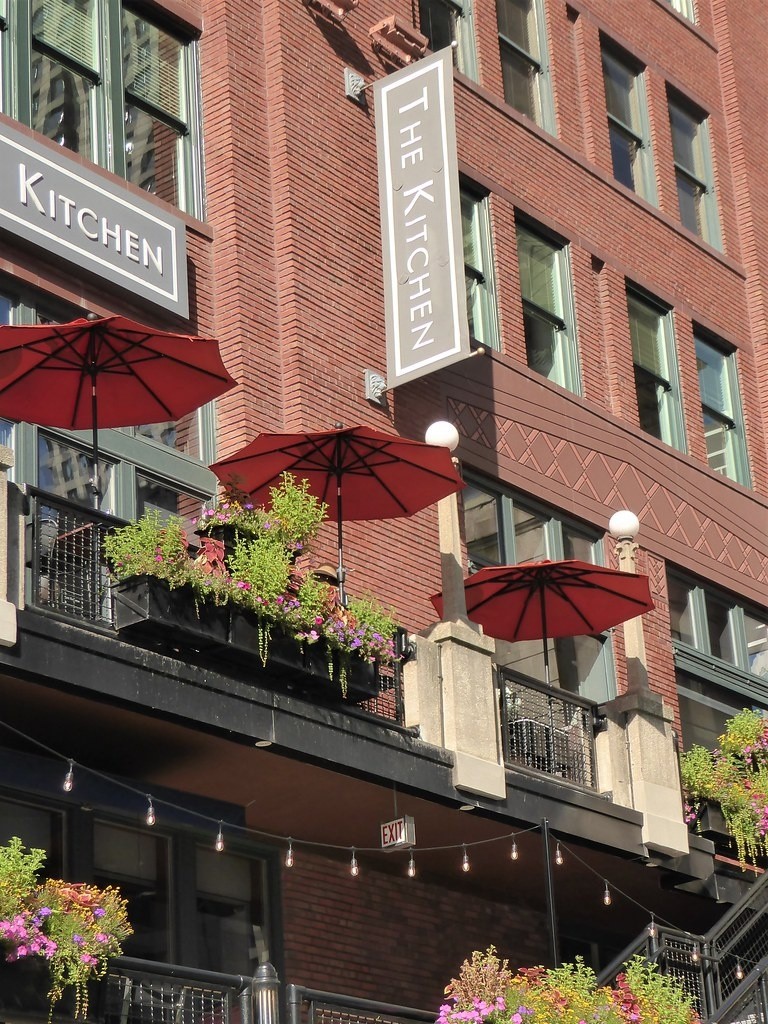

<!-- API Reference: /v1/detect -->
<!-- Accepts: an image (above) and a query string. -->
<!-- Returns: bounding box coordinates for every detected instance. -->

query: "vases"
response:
[0,939,108,1024]
[107,572,380,705]
[684,795,766,853]
[193,523,302,566]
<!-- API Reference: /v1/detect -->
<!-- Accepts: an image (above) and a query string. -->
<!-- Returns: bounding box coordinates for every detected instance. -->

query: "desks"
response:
[501,719,568,778]
[56,523,114,626]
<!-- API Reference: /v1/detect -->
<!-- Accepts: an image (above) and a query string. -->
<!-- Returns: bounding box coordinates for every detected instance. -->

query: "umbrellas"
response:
[0,312,237,613]
[206,422,468,604]
[428,558,656,772]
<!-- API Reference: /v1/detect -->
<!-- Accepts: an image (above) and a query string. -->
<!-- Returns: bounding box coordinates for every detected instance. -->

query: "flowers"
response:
[680,707,768,879]
[102,510,401,700]
[433,943,701,1024]
[0,836,133,1024]
[191,473,330,552]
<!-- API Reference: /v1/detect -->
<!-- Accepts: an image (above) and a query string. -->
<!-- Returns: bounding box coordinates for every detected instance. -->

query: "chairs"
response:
[25,518,94,618]
